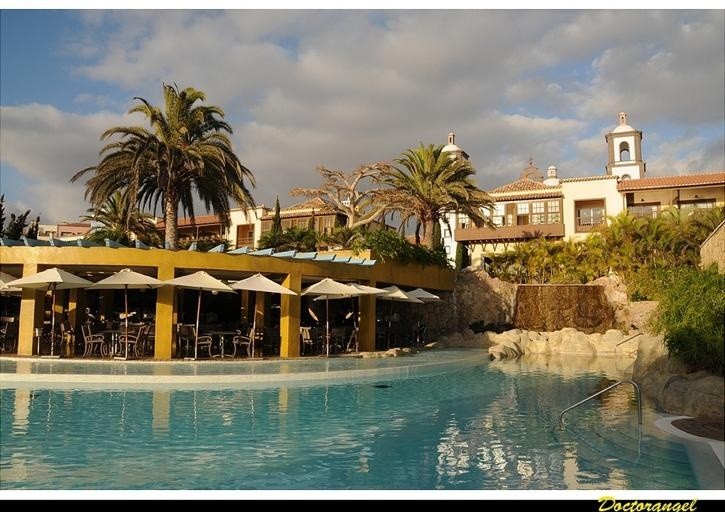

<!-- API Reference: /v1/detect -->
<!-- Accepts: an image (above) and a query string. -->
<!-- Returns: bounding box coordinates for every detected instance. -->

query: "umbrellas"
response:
[5,267,95,355]
[313,282,388,352]
[86,268,167,358]
[163,270,238,359]
[377,285,425,346]
[300,277,364,357]
[228,272,298,358]
[406,288,444,342]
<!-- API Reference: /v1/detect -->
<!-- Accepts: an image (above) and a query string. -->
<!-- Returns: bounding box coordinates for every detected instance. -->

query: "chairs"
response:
[0,320,427,358]
